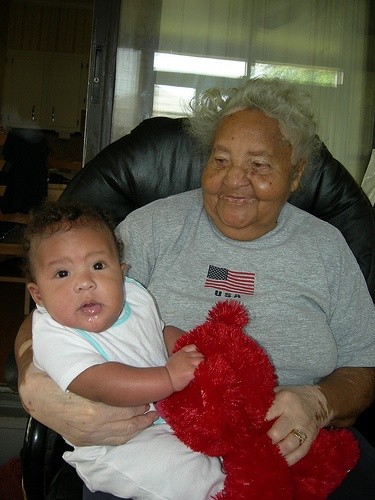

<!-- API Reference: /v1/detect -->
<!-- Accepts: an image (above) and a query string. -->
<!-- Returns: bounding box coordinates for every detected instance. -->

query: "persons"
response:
[13,73,375,500]
[22,201,231,500]
[48,130,82,162]
[0,125,50,246]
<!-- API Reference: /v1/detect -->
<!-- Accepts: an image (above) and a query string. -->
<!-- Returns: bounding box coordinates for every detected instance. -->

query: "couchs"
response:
[21,115,375,500]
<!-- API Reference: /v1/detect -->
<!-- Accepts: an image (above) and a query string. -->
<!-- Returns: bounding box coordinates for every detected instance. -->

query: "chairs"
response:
[0,183,67,316]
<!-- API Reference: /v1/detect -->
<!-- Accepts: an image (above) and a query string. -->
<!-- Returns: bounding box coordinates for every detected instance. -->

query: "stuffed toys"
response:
[152,300,361,500]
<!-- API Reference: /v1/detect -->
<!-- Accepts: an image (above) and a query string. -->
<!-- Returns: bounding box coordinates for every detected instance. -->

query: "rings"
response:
[291,429,307,446]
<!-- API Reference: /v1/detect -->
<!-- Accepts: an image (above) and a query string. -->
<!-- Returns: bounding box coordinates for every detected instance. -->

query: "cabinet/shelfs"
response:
[0,50,83,140]
